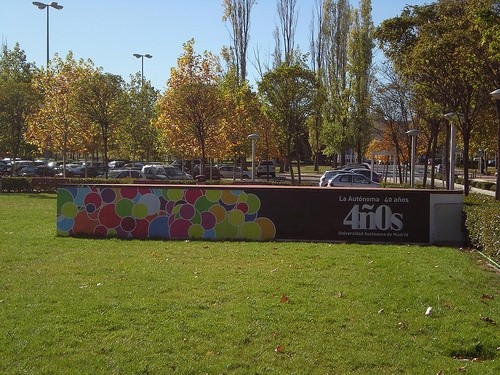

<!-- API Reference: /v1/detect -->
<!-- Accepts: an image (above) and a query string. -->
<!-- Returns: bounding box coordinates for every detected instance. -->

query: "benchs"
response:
[32,177,69,193]
[132,178,170,185]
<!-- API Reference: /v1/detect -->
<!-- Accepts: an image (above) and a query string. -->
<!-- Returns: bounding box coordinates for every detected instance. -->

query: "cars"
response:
[418,145,497,165]
[214,164,252,179]
[319,162,382,188]
[0,158,221,180]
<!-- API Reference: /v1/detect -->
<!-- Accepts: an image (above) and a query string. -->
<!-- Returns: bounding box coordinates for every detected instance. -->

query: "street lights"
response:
[482,139,488,171]
[406,130,420,188]
[133,54,152,111]
[444,112,464,191]
[248,133,260,182]
[32,2,63,90]
[489,88,500,200]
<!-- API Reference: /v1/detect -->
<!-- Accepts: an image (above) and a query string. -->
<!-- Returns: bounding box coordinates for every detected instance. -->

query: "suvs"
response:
[255,160,276,178]
